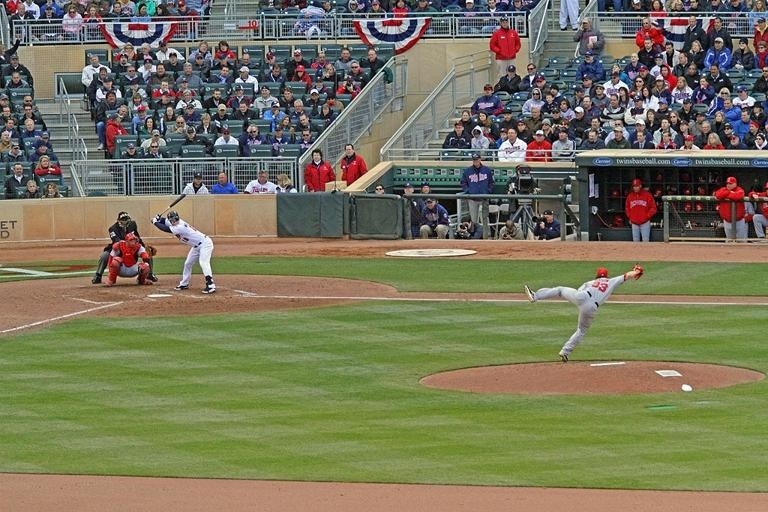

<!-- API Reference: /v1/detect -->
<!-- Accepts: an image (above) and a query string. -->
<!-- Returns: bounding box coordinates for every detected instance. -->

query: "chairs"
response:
[439,54,767,161]
[475,203,581,236]
[84,44,396,192]
[3,1,210,46]
[253,0,536,37]
[2,76,68,199]
[598,1,768,37]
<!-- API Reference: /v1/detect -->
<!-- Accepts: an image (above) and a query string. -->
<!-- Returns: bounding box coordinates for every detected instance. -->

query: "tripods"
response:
[510,198,538,239]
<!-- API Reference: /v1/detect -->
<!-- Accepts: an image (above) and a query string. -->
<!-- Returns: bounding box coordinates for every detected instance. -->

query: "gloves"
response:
[142,253,151,261]
[112,243,119,251]
[151,218,159,224]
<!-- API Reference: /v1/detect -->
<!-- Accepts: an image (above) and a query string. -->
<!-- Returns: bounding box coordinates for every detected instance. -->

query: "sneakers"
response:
[201,285,216,293]
[147,273,158,282]
[92,276,102,284]
[137,278,154,285]
[173,285,190,291]
[524,285,536,303]
[105,279,116,289]
[559,350,568,362]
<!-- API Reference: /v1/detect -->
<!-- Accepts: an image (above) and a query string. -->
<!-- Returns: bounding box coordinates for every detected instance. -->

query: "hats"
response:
[118,212,131,220]
[372,0,381,6]
[472,154,481,160]
[585,51,593,56]
[726,177,736,184]
[98,53,336,150]
[653,53,663,59]
[178,0,185,6]
[597,268,609,279]
[582,17,591,24]
[46,4,53,9]
[159,41,166,45]
[466,0,474,5]
[194,173,202,178]
[11,54,18,59]
[544,210,552,215]
[632,178,642,187]
[403,181,435,202]
[500,17,508,22]
[757,17,766,23]
[455,64,767,141]
[715,37,723,44]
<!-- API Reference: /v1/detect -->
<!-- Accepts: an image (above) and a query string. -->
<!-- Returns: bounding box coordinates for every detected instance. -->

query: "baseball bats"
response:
[160,194,185,216]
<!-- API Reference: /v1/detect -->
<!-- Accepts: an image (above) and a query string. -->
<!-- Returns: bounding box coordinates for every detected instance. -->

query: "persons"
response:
[102,233,153,287]
[151,211,216,294]
[91,211,157,284]
[524,263,644,363]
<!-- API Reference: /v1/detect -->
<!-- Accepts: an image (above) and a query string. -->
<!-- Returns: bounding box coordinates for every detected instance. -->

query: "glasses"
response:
[68,11,75,14]
[179,4,184,8]
[1,95,48,150]
[46,8,52,10]
[160,44,167,48]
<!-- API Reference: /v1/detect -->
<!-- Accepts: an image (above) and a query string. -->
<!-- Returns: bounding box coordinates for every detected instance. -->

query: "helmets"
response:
[167,210,179,223]
[125,233,139,249]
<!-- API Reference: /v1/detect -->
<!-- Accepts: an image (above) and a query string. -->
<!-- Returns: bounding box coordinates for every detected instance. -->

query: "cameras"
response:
[503,232,509,238]
[460,224,468,229]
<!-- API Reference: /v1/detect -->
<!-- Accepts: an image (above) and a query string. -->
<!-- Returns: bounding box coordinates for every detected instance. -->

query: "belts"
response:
[194,234,207,248]
[588,291,600,308]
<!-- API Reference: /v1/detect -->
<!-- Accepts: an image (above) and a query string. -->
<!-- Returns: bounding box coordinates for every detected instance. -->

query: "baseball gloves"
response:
[146,244,157,257]
[633,263,644,280]
